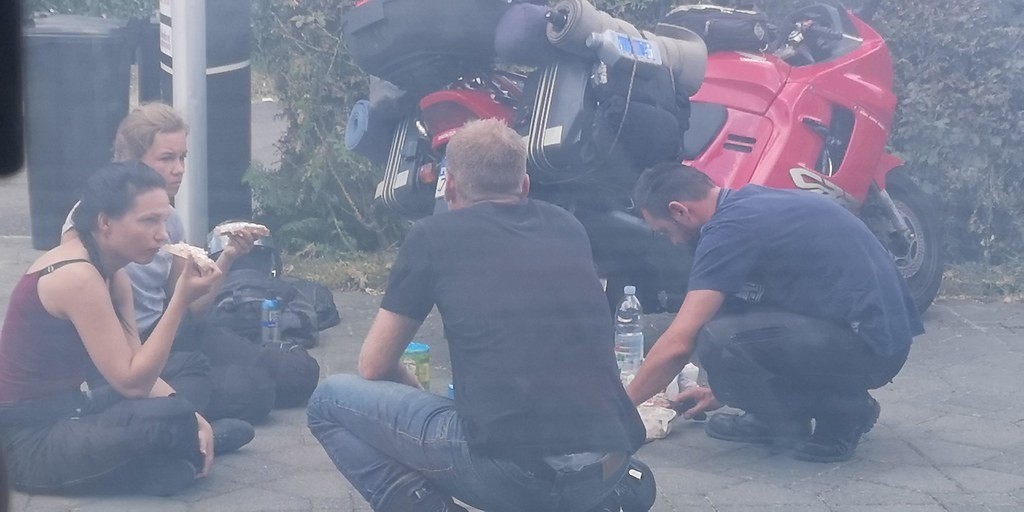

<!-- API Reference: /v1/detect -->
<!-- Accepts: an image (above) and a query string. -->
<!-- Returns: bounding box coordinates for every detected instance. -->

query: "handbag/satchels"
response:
[208,267,319,351]
[261,338,320,407]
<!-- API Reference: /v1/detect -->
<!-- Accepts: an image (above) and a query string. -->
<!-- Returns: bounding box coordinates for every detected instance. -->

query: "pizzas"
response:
[214,221,269,237]
[162,243,210,271]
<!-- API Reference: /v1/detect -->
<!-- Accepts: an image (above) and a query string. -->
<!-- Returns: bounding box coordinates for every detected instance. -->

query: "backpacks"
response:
[663,4,771,53]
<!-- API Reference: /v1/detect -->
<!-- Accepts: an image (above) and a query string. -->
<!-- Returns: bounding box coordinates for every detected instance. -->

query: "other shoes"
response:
[704,412,813,442]
[209,418,255,455]
[130,460,195,496]
[795,392,881,462]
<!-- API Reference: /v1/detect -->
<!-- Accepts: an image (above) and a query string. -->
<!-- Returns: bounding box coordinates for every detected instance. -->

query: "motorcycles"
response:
[334,0,948,317]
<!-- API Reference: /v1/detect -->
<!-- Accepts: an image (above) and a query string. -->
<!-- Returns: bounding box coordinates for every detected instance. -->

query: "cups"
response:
[398,343,431,392]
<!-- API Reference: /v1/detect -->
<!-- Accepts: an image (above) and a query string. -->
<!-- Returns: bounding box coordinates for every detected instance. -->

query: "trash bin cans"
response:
[15,8,149,259]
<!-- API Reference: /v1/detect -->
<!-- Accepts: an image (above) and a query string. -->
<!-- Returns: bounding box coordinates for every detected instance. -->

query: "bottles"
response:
[585,30,662,78]
[615,285,643,369]
[262,290,281,343]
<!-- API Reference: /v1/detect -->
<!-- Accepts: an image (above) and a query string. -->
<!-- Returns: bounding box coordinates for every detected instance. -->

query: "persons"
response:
[1,101,320,498]
[626,160,925,458]
[314,120,649,512]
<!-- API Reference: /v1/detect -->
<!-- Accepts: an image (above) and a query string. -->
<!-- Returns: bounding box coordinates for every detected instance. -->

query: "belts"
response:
[510,450,630,482]
